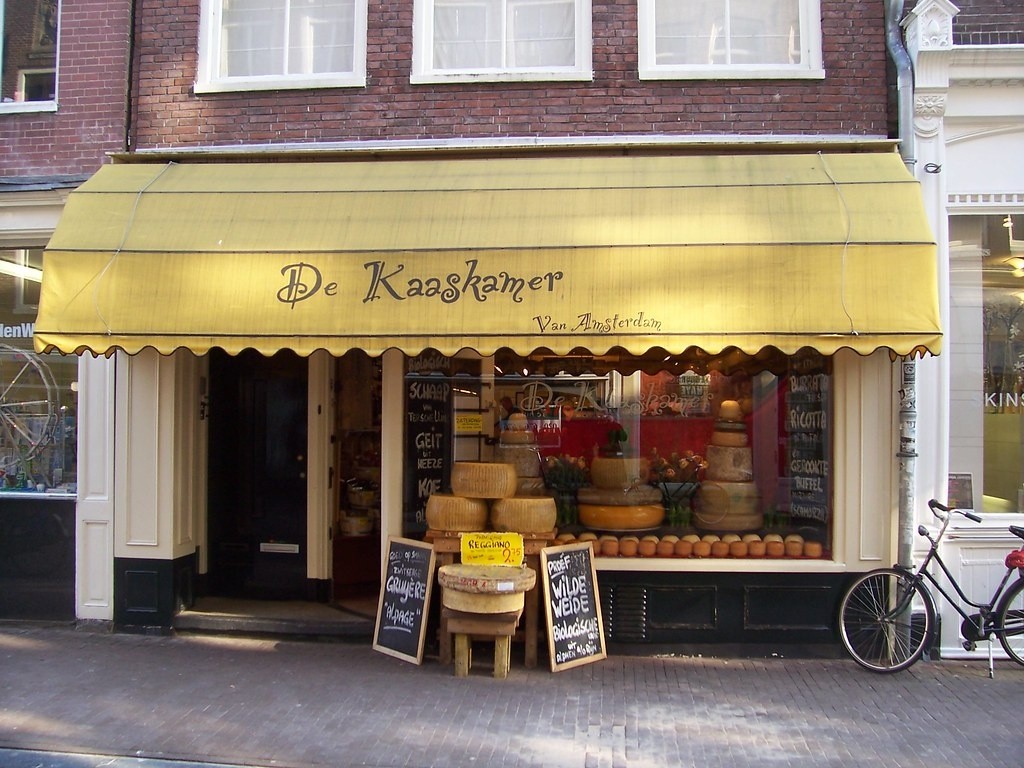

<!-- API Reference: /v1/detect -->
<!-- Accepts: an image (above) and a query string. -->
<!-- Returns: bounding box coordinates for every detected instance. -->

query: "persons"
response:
[500,397,521,420]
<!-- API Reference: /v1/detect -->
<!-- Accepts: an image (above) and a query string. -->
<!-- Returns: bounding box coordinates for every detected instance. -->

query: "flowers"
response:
[543,417,709,502]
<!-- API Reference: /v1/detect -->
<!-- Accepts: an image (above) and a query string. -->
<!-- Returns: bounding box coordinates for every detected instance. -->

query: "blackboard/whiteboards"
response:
[371,534,438,667]
[539,540,608,672]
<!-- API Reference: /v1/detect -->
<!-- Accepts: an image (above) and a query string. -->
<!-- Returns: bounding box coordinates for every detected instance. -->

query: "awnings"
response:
[31,152,945,358]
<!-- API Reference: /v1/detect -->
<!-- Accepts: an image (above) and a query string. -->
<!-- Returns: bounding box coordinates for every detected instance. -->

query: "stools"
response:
[448,607,523,680]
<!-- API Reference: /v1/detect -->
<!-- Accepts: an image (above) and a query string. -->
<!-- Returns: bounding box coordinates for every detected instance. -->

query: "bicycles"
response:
[626,458,730,525]
[837,498,1024,679]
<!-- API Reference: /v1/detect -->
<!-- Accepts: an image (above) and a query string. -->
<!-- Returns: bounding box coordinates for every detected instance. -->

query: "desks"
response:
[425,530,556,671]
